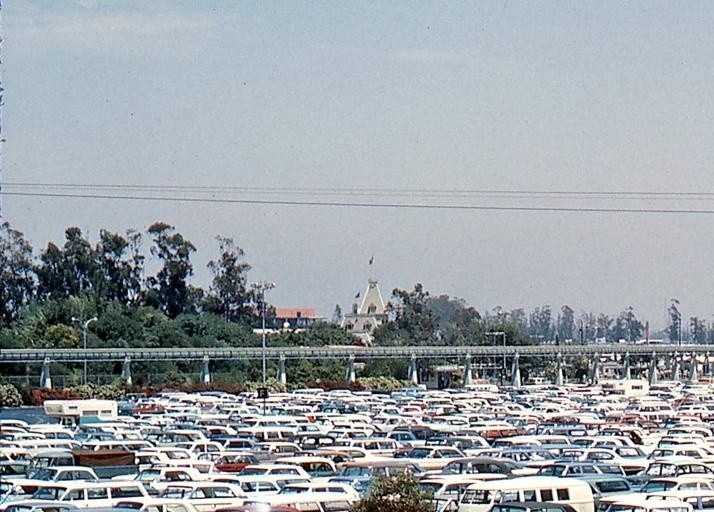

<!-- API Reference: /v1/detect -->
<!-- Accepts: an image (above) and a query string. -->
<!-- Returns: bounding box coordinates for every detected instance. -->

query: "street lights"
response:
[503,311,512,370]
[71,316,98,385]
[251,280,276,385]
